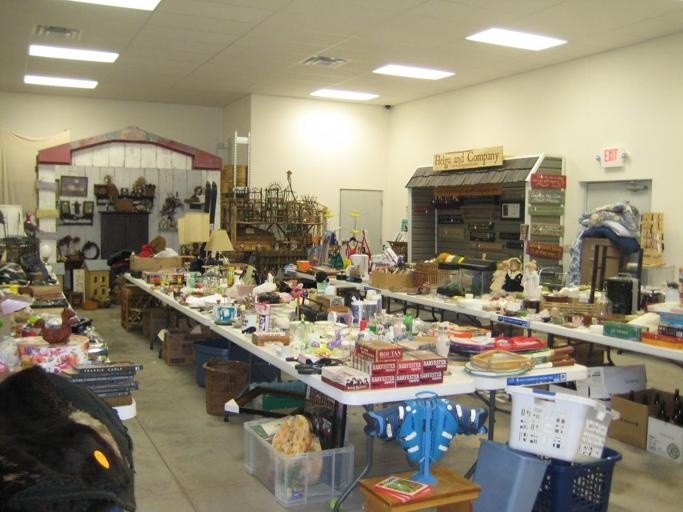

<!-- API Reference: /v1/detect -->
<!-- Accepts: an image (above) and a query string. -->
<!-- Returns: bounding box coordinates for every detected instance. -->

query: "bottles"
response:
[403,310,413,339]
[227,266,234,286]
[664,282,678,303]
[154,275,160,286]
[350,266,360,278]
[371,269,412,292]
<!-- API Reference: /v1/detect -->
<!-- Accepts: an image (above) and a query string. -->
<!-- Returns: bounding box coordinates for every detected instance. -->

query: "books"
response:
[59,361,144,398]
[638,324,683,353]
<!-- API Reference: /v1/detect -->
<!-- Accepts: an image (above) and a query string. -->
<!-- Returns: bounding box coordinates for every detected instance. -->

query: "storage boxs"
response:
[501,439,625,512]
[506,384,621,463]
[162,331,203,366]
[646,414,683,460]
[614,389,683,451]
[575,364,647,400]
[193,336,282,388]
[244,417,357,505]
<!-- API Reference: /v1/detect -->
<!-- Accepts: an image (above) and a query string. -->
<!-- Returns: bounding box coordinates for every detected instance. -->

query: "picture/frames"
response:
[61,201,69,215]
[83,201,93,217]
[60,175,88,196]
[500,200,522,221]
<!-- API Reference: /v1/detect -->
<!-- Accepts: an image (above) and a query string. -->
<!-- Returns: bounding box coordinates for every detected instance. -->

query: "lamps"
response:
[204,226,232,264]
[39,242,53,265]
[178,208,210,259]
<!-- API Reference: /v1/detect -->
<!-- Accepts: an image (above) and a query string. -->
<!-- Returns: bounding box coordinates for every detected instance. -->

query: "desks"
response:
[123,266,585,512]
[1,263,136,430]
[297,259,683,365]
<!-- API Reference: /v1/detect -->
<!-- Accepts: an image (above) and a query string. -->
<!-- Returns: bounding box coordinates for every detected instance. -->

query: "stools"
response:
[354,468,483,512]
